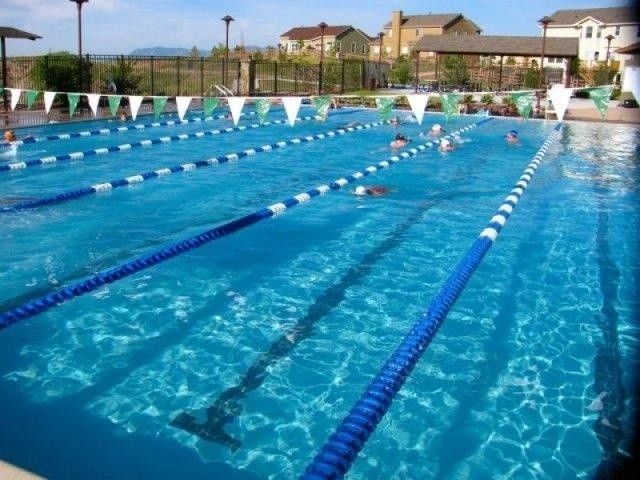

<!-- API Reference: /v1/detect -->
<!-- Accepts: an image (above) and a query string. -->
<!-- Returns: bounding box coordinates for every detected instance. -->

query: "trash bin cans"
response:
[368,76,375,90]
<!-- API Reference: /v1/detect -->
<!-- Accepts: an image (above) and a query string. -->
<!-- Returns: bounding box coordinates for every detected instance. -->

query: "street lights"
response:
[317,22,328,96]
[69,0,89,114]
[220,15,234,88]
[376,32,386,88]
[537,16,554,89]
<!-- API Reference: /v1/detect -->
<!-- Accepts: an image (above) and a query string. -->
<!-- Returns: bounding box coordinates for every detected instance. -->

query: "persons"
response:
[505,130,520,142]
[3,129,16,141]
[391,115,401,126]
[438,138,456,151]
[120,111,129,121]
[419,123,445,137]
[390,134,412,150]
[361,184,390,196]
[331,97,341,109]
